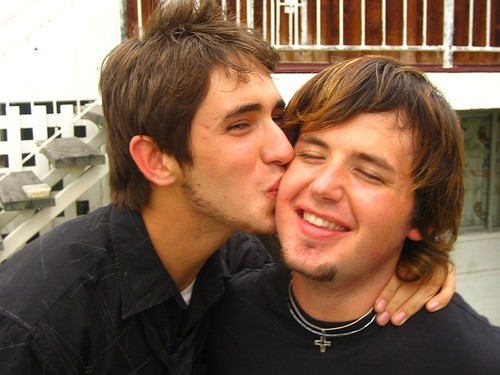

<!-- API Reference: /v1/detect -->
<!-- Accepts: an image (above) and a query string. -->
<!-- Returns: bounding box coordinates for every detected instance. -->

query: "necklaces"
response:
[285,269,379,354]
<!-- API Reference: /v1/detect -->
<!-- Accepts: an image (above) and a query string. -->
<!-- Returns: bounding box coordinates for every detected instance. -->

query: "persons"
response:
[190,56,500,375]
[0,1,455,375]
